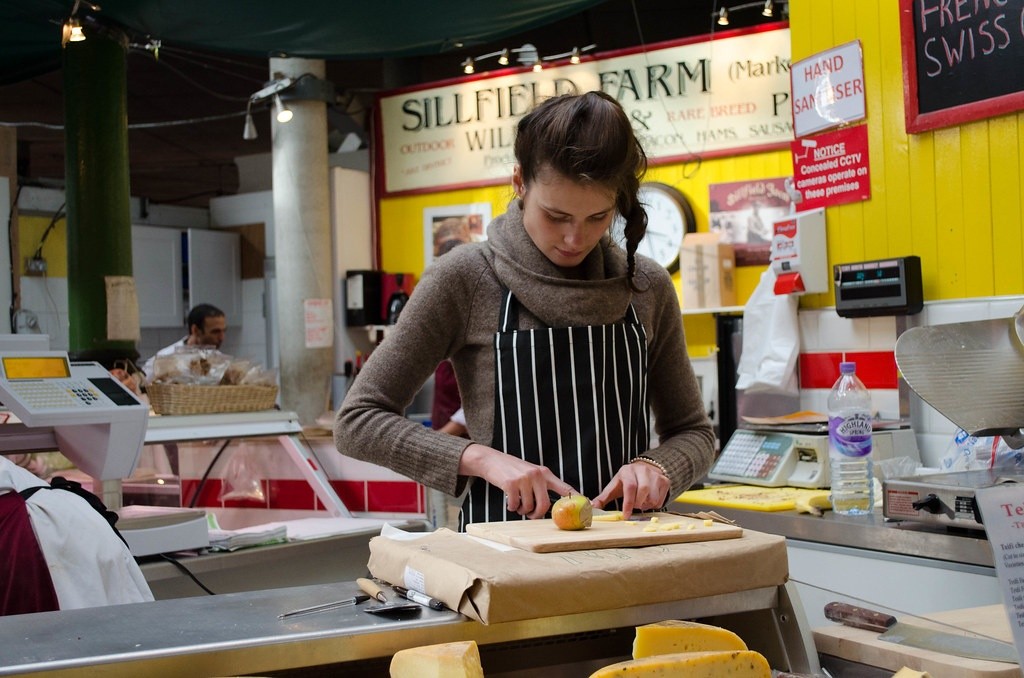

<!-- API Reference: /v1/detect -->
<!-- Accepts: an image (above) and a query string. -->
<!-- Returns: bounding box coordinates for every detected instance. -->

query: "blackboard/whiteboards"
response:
[899,0,1024,134]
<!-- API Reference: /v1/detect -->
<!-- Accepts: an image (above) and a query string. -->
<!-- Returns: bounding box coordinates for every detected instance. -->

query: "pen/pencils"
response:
[278,596,370,620]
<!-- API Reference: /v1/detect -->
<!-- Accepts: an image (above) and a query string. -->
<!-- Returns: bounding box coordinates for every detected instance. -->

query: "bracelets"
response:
[629,457,668,477]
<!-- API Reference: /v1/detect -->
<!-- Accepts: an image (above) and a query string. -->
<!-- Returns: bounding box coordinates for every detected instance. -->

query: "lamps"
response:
[69,17,86,42]
[243,102,258,140]
[464,57,475,74]
[718,8,729,25]
[272,93,294,123]
[762,0,774,16]
[534,60,542,72]
[570,47,580,64]
[498,48,510,64]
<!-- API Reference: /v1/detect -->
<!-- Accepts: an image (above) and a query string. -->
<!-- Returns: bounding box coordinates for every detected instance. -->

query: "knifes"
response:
[504,493,608,516]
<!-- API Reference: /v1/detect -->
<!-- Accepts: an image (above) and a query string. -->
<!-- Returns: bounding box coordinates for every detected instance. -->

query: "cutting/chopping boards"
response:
[671,483,841,512]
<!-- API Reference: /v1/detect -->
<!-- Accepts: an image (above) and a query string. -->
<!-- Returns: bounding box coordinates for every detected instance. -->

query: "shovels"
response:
[356,578,422,613]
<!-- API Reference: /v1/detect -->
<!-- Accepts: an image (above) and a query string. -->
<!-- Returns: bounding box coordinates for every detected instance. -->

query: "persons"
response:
[334,89,717,533]
[142,303,228,385]
[429,357,472,440]
[0,454,155,617]
[746,203,772,246]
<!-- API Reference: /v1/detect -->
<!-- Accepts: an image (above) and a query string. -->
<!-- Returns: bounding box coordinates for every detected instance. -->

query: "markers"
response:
[392,586,444,611]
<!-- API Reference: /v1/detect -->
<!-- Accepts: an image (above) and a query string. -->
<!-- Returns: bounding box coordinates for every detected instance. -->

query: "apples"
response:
[552,493,589,530]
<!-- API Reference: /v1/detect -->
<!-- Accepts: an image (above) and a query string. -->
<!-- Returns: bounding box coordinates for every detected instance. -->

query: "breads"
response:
[151,355,254,385]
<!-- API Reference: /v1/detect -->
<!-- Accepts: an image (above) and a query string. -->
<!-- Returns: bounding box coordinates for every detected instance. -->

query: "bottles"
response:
[826,360,875,516]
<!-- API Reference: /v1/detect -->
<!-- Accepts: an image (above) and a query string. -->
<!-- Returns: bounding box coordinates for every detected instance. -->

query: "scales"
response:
[707,422,921,490]
[0,333,210,562]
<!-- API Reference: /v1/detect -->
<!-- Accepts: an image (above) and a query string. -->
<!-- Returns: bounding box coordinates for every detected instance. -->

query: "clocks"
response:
[605,182,698,275]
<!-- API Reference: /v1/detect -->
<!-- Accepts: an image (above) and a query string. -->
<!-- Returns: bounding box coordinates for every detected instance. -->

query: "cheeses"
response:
[632,619,748,659]
[588,650,773,678]
[389,640,484,678]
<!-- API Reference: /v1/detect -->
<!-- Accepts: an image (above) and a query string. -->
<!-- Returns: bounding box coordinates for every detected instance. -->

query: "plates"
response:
[466,510,744,553]
